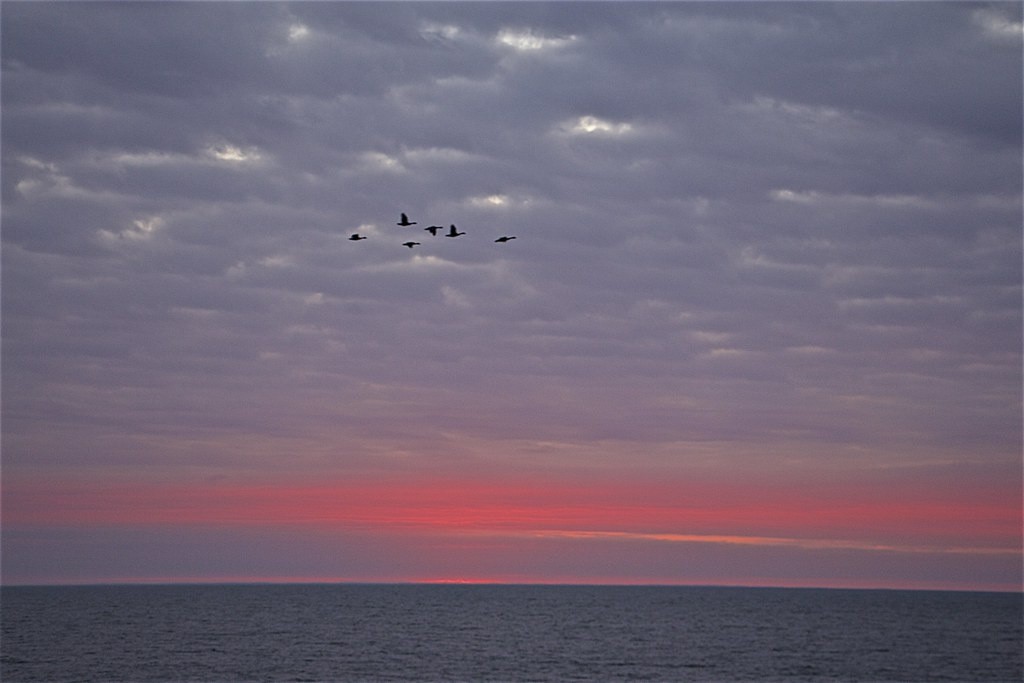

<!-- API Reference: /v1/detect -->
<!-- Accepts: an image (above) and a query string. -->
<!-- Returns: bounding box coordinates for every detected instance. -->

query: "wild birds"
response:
[397,212,417,226]
[445,224,466,237]
[494,237,516,242]
[424,226,443,236]
[349,234,367,240]
[402,241,420,248]
